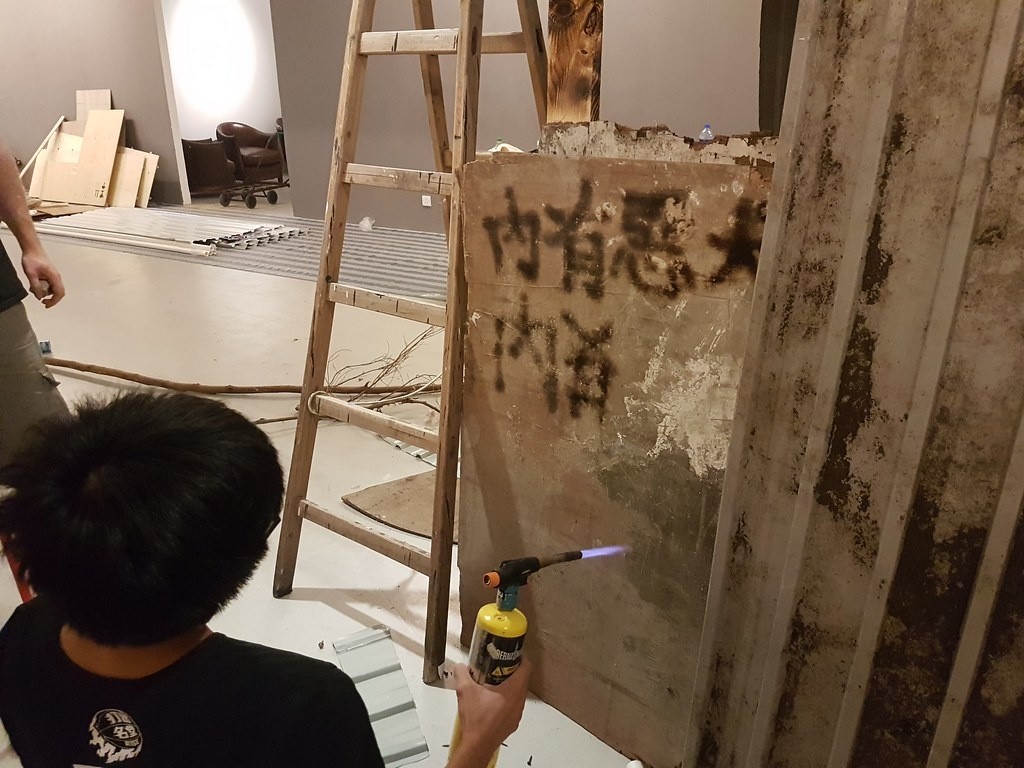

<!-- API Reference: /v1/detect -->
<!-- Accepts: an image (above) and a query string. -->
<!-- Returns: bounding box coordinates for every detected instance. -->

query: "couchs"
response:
[216,121,284,185]
[182,138,237,194]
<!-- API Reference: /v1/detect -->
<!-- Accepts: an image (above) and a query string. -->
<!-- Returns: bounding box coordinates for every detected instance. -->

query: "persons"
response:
[1,381,533,768]
[0,128,77,486]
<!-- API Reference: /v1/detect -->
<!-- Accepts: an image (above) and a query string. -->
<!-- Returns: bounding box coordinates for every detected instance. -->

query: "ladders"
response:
[273,0,549,682]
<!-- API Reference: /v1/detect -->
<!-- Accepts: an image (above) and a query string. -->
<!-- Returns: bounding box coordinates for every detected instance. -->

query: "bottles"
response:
[698,124,715,143]
